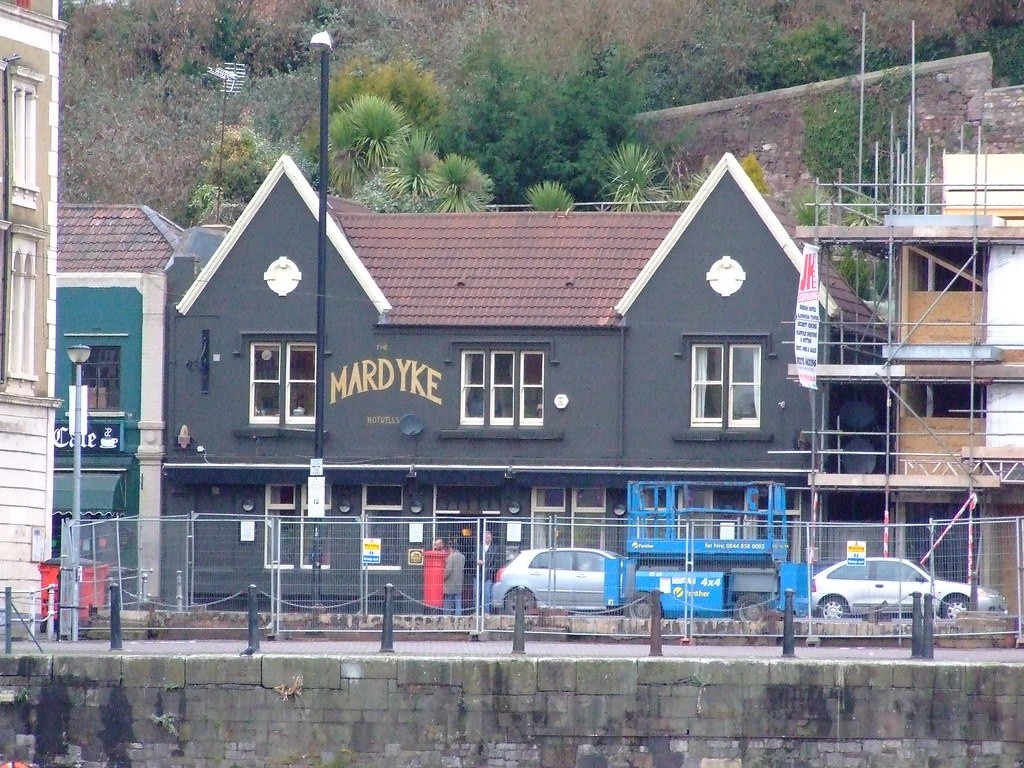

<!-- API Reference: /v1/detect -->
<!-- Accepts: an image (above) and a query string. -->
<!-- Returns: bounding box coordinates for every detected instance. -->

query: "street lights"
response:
[310,30,333,612]
[68,345,90,641]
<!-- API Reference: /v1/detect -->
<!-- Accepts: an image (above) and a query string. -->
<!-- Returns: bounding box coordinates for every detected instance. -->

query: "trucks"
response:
[603,480,813,620]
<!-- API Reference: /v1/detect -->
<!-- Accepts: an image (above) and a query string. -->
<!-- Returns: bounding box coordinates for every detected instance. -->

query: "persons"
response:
[435,539,465,615]
[472,530,496,615]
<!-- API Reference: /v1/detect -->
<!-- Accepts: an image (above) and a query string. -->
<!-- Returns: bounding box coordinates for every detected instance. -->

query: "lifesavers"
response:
[1,761,30,768]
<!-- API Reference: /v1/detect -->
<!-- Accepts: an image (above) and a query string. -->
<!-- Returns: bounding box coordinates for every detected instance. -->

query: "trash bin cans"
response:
[38,557,111,633]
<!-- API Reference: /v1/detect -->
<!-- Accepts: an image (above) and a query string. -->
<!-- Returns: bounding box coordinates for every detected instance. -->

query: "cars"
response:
[812,557,1007,619]
[491,547,627,614]
